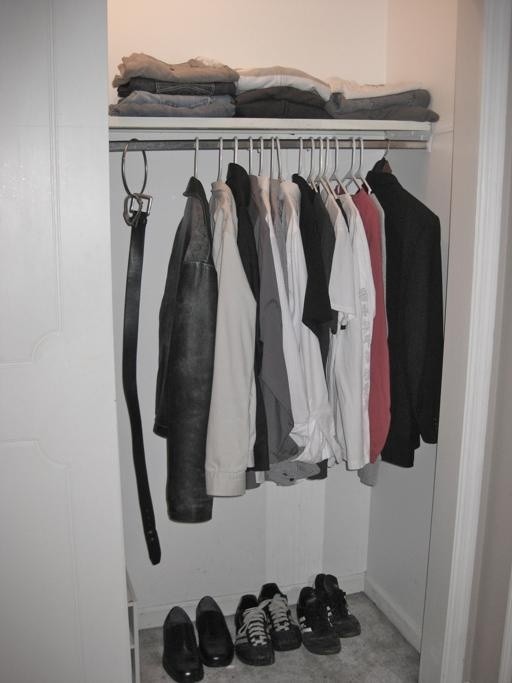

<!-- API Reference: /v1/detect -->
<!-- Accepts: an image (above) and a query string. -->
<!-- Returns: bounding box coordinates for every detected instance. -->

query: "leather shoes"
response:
[195,595,235,668]
[161,605,204,683]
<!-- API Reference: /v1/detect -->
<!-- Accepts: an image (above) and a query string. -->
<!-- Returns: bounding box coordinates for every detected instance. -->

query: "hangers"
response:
[176,138,442,263]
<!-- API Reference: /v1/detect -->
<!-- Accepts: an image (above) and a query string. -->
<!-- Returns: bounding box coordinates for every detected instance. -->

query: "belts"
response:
[119,190,161,567]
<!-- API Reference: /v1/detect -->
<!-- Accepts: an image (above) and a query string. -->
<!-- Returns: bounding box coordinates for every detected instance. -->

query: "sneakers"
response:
[234,582,302,667]
[294,572,361,655]
